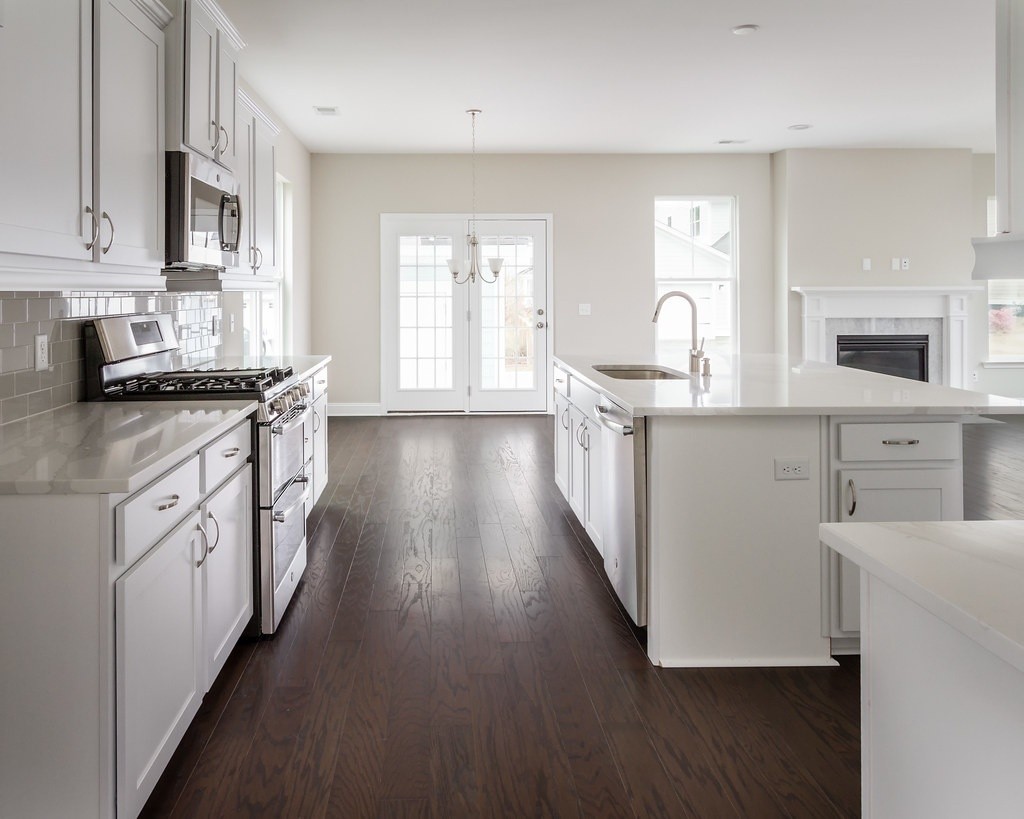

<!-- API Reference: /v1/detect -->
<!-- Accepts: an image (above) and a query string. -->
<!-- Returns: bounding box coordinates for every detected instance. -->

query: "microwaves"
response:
[165,151,243,267]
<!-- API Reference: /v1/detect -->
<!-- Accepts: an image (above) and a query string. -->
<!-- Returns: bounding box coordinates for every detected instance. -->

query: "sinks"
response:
[592,363,691,379]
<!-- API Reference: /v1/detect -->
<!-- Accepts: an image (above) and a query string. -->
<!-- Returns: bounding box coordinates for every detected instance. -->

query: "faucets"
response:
[651,289,701,372]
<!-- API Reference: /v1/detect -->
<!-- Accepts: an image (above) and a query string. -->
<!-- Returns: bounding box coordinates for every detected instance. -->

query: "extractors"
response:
[162,272,280,292]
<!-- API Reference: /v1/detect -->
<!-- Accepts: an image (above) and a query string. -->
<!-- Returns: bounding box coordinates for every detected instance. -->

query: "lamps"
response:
[444,110,505,284]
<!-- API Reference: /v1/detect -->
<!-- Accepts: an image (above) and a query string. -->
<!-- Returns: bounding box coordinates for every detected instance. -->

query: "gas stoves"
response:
[84,314,310,423]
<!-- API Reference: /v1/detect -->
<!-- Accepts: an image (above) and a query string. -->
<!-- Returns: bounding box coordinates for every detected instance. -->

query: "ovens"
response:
[252,397,308,636]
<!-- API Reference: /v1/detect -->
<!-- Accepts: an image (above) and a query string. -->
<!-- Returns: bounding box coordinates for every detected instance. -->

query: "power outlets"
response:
[33,334,47,372]
[775,457,810,480]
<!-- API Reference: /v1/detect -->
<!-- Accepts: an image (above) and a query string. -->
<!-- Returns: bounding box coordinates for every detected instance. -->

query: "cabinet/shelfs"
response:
[160,85,285,292]
[311,365,327,506]
[553,362,568,504]
[568,374,603,559]
[0,0,172,292]
[300,377,314,519]
[1,419,252,819]
[822,413,964,655]
[157,0,245,176]
